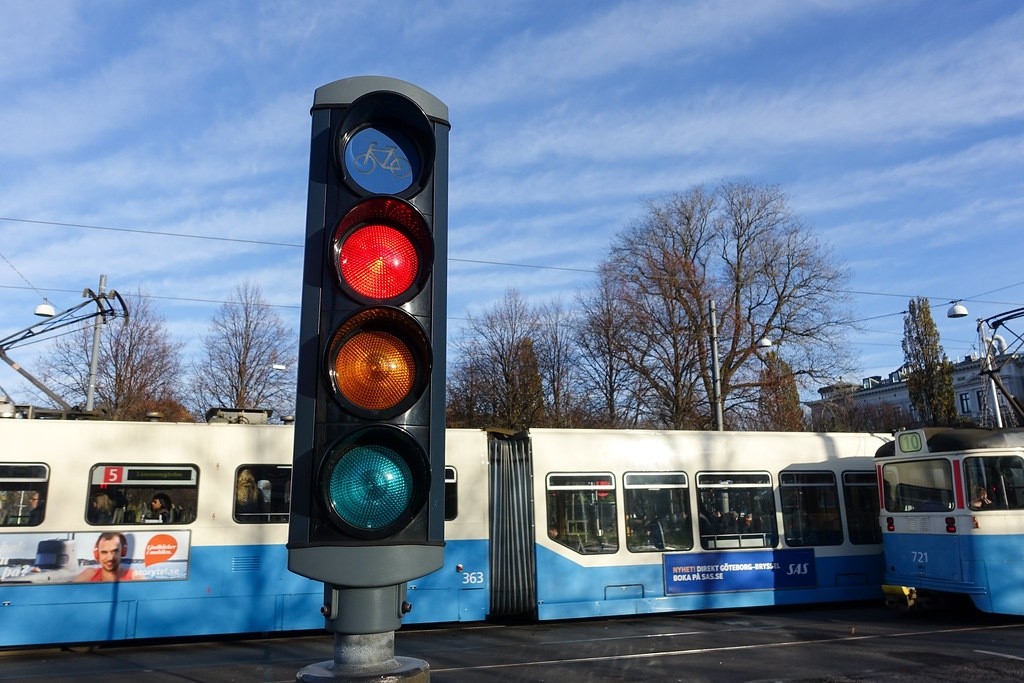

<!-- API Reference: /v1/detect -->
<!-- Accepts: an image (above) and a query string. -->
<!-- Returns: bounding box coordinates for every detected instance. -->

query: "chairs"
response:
[108,501,186,525]
[649,519,672,549]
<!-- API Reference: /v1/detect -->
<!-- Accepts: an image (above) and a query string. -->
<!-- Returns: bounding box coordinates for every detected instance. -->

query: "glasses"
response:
[30,497,41,501]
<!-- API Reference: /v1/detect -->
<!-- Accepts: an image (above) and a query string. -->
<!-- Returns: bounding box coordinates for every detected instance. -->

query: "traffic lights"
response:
[283,76,453,589]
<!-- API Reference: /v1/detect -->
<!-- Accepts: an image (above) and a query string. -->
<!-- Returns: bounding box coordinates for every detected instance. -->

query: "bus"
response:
[873,426,1024,620]
[0,381,898,657]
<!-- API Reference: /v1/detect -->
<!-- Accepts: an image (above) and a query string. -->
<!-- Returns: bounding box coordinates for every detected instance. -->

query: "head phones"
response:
[94,532,127,564]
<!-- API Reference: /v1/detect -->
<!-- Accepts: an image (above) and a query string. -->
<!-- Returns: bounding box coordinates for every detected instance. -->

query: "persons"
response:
[24,490,45,527]
[87,486,129,524]
[142,493,172,524]
[70,531,143,582]
